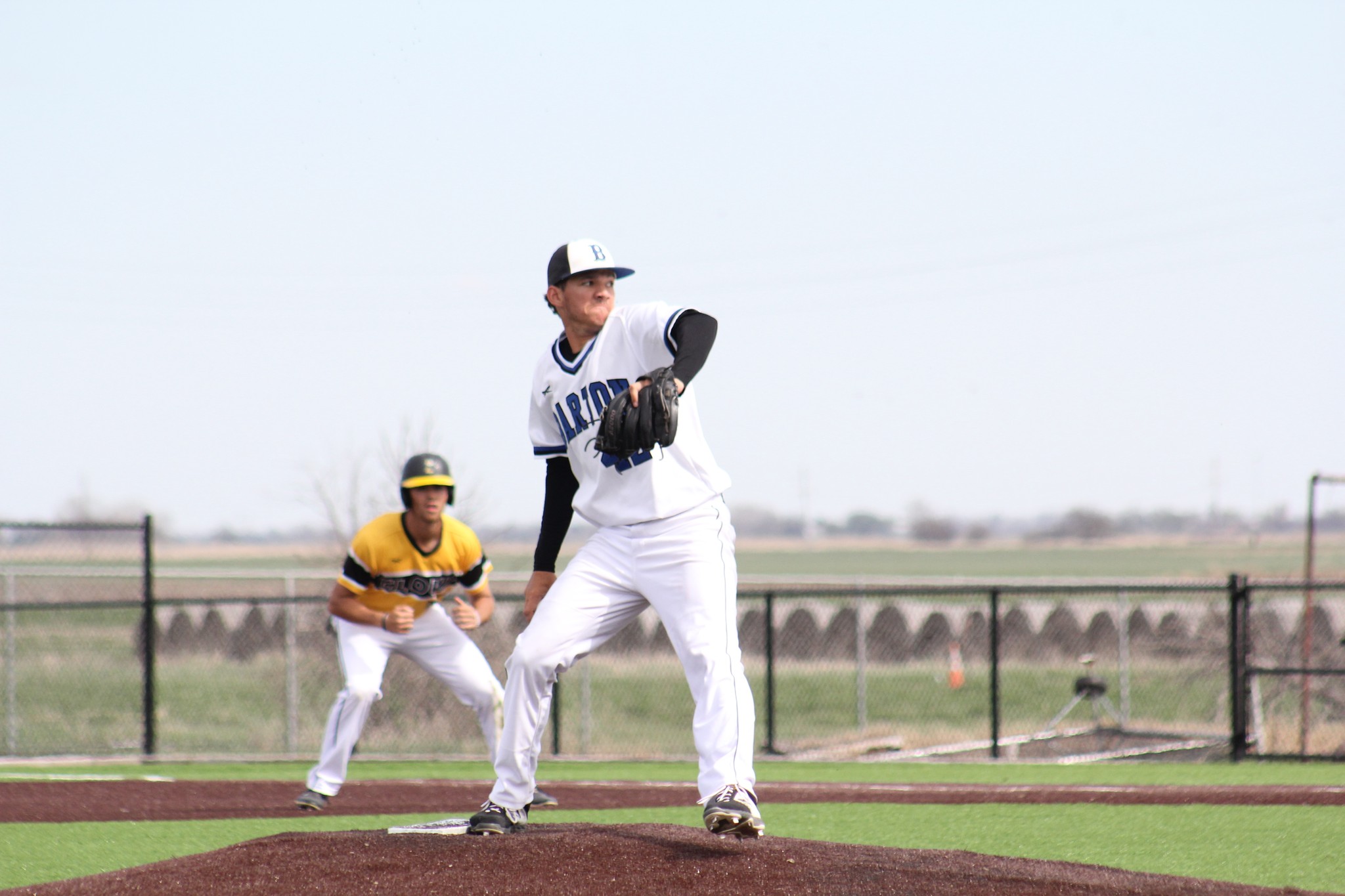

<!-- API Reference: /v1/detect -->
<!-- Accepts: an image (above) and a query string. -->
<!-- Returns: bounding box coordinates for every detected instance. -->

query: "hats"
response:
[547,240,635,285]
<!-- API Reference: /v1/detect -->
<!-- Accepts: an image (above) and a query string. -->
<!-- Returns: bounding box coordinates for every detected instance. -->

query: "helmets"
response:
[400,454,454,488]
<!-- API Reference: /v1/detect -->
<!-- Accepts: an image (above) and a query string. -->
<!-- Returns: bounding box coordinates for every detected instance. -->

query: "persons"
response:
[466,241,766,843]
[295,453,558,811]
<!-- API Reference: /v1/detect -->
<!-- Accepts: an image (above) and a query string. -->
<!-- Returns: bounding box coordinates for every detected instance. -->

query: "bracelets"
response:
[382,614,389,631]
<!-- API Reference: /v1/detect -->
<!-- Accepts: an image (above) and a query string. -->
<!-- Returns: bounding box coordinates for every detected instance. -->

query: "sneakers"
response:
[293,791,327,811]
[531,791,559,808]
[703,784,764,838]
[471,801,529,835]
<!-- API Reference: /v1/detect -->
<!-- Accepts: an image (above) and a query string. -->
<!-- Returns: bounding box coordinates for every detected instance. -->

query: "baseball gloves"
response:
[584,363,678,475]
